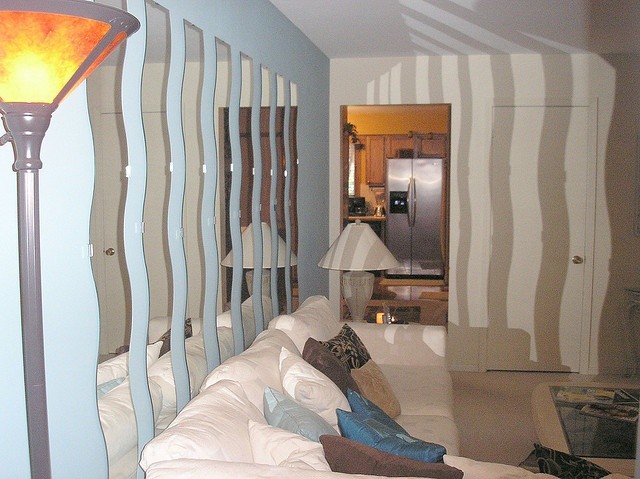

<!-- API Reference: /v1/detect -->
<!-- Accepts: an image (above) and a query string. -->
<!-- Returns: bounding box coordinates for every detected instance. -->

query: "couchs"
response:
[96,296,272,477]
[140,296,634,477]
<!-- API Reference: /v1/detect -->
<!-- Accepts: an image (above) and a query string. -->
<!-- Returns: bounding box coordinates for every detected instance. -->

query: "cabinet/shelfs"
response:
[387,133,447,158]
[365,134,386,187]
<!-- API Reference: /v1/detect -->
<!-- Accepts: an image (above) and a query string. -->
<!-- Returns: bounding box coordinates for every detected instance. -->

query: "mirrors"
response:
[260,64,273,331]
[141,1,177,438]
[275,73,287,315]
[181,19,208,399]
[86,0,139,479]
[213,35,235,365]
[239,52,256,351]
[289,82,299,313]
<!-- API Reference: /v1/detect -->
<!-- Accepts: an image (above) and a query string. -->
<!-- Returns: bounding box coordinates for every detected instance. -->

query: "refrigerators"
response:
[386,159,445,277]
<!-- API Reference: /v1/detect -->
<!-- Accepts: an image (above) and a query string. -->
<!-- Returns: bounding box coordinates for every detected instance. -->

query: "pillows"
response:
[263,385,339,444]
[279,345,352,436]
[96,340,162,380]
[336,408,446,463]
[248,418,333,471]
[317,323,371,369]
[531,442,612,478]
[151,317,193,359]
[347,387,409,436]
[350,358,401,419]
[302,337,360,397]
[319,435,464,479]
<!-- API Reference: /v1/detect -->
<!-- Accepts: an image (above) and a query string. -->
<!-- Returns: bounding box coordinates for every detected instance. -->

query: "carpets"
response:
[344,305,421,324]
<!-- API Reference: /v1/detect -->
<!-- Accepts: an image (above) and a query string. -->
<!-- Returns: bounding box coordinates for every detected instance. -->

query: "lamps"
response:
[317,219,401,322]
[220,221,297,297]
[0,1,141,479]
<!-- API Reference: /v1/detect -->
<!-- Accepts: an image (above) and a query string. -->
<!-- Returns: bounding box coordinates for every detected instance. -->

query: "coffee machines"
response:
[349,197,366,216]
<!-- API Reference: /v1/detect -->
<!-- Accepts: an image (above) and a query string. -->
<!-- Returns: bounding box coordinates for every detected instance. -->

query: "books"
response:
[555,386,615,407]
[579,403,640,423]
